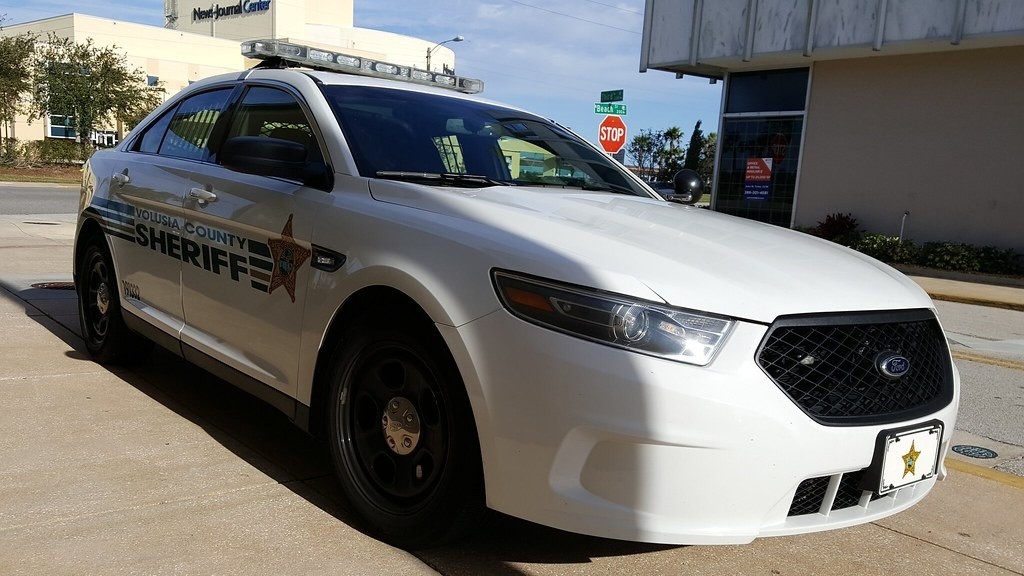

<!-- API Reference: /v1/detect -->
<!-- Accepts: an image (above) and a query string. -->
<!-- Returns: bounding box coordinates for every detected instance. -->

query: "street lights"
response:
[426,35,464,73]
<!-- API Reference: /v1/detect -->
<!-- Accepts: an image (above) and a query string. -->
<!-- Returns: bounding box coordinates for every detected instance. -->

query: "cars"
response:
[73,41,962,546]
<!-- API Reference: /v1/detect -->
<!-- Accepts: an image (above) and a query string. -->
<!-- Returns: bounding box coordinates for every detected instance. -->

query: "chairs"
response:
[268,128,313,148]
[357,110,448,174]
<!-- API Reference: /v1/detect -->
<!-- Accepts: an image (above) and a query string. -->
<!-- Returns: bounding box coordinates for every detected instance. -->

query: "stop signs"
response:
[599,114,626,153]
